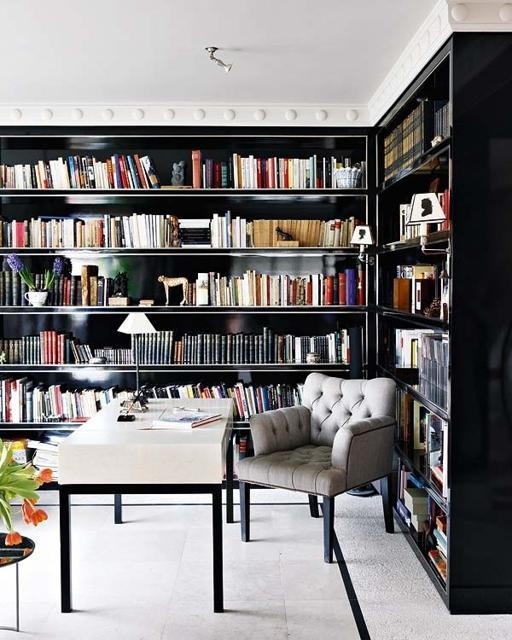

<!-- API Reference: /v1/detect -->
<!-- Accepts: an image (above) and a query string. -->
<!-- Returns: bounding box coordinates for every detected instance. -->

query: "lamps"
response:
[348,225,376,267]
[117,312,157,405]
[404,191,451,256]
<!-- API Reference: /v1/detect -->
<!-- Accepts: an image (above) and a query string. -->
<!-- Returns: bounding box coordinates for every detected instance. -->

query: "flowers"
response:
[6,253,67,292]
[1,440,55,546]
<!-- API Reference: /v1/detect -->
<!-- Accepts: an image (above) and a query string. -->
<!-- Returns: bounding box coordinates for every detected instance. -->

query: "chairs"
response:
[235,370,396,563]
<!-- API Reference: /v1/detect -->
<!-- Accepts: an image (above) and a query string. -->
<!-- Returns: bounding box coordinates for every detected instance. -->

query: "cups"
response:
[25,292,49,306]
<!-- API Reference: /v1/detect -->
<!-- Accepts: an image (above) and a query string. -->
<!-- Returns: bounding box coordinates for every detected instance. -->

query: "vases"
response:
[332,168,361,188]
[23,291,48,307]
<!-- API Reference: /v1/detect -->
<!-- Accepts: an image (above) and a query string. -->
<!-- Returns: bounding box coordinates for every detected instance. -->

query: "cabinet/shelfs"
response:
[373,31,512,618]
[0,126,373,488]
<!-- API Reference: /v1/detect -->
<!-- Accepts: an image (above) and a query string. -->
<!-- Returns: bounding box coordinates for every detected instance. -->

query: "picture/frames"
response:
[412,400,431,450]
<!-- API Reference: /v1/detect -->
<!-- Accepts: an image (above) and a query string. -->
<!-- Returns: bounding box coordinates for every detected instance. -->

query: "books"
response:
[154,413,221,428]
[1,213,182,248]
[0,265,114,306]
[238,437,247,453]
[31,430,74,480]
[0,154,159,189]
[1,377,304,422]
[1,326,350,364]
[210,210,362,247]
[382,98,450,576]
[193,150,367,188]
[186,269,358,306]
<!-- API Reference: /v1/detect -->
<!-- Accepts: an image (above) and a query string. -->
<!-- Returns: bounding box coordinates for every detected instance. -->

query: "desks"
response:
[57,396,235,612]
